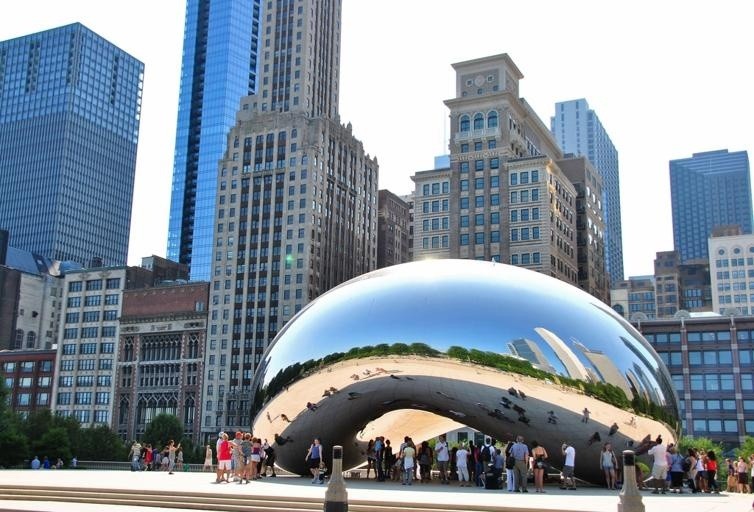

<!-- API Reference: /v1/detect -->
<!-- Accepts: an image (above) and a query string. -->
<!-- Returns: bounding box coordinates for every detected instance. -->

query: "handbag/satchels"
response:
[505,457,515,468]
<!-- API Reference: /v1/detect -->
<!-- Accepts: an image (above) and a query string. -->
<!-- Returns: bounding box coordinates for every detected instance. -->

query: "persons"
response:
[316,461,328,484]
[127,440,183,474]
[390,374,400,379]
[647,437,720,494]
[635,462,650,489]
[71,455,77,468]
[588,431,601,447]
[347,397,361,400]
[30,455,40,469]
[328,386,339,394]
[42,455,50,468]
[273,432,291,445]
[608,422,619,435]
[304,434,322,483]
[449,408,476,418]
[348,391,361,398]
[436,391,453,400]
[350,366,387,381]
[599,440,619,489]
[280,413,291,423]
[546,409,558,424]
[321,389,331,398]
[634,434,662,455]
[487,387,531,426]
[215,430,277,484]
[560,442,576,489]
[266,411,272,423]
[725,454,754,493]
[305,401,317,412]
[379,397,410,407]
[582,407,590,422]
[476,401,488,412]
[405,376,415,380]
[410,401,440,414]
[55,457,63,468]
[202,444,213,472]
[626,441,633,449]
[365,432,548,492]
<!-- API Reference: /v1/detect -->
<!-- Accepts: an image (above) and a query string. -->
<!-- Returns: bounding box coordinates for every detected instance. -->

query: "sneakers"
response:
[260,472,267,477]
[366,476,577,493]
[271,473,276,477]
[606,486,721,495]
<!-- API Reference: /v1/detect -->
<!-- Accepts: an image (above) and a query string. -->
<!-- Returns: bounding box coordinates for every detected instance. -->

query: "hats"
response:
[235,432,241,438]
[218,431,224,437]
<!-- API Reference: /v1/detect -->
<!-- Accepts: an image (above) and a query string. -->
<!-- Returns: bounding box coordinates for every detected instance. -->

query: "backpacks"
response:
[480,447,491,461]
[679,457,691,472]
